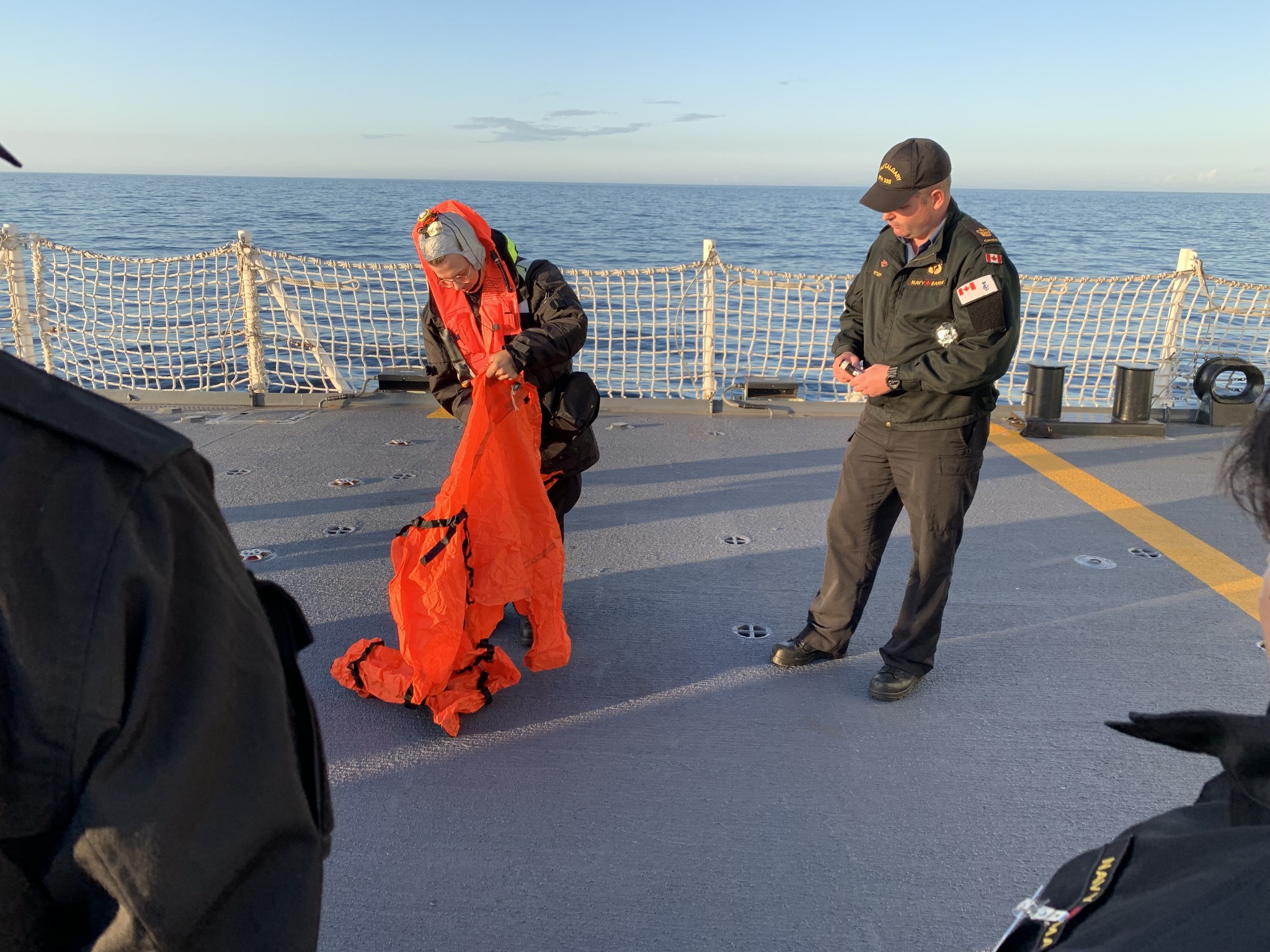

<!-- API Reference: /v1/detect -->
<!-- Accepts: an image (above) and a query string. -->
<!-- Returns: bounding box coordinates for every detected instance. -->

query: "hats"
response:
[418,212,486,270]
[860,137,952,213]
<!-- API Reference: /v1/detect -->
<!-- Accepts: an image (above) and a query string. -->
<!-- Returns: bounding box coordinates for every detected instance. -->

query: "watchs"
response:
[885,365,903,392]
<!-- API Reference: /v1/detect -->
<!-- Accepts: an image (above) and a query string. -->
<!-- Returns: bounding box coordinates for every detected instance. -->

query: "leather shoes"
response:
[770,634,845,667]
[867,664,925,699]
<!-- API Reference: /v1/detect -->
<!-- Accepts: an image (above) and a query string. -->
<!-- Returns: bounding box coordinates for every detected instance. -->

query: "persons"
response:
[419,211,600,647]
[0,347,337,952]
[770,139,1020,702]
[993,384,1270,952]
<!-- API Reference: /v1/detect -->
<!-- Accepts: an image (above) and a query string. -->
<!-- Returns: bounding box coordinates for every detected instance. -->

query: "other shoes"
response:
[519,615,533,645]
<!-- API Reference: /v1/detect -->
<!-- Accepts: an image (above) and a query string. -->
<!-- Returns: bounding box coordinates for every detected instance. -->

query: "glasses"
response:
[436,264,472,288]
